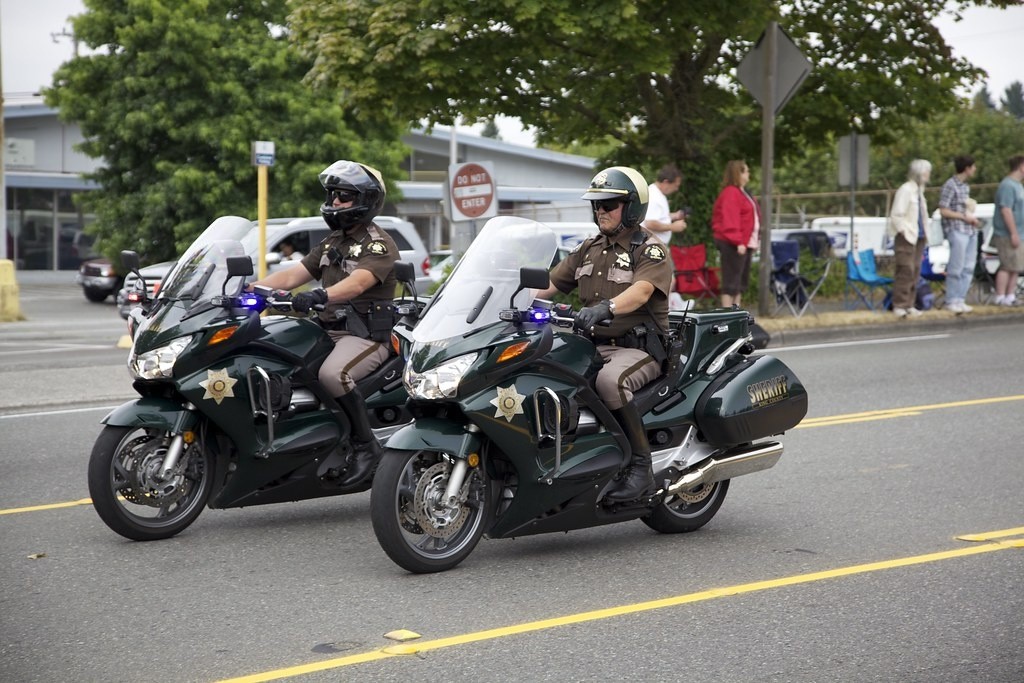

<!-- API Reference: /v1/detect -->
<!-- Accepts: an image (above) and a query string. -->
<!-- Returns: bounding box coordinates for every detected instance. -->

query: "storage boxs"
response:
[692,353,807,449]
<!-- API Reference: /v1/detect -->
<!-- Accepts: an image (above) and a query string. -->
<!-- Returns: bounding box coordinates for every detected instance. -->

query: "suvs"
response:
[119,214,429,320]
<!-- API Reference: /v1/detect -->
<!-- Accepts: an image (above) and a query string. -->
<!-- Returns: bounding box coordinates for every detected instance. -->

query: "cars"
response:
[76,257,116,302]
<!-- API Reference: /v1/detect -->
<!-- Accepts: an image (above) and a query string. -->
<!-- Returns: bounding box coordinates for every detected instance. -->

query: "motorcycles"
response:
[371,214,809,573]
[86,214,427,540]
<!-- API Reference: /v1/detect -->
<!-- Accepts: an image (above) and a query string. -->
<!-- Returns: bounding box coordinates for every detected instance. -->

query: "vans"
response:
[810,204,1001,276]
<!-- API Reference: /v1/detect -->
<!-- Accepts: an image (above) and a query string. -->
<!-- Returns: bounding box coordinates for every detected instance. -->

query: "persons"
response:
[890,160,932,317]
[241,160,401,488]
[278,241,305,263]
[990,154,1024,306]
[712,161,760,309]
[640,167,687,248]
[467,166,674,502]
[940,156,983,313]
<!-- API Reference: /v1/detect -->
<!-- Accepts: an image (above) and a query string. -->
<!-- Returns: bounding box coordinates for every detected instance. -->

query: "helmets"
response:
[581,166,650,237]
[318,160,386,231]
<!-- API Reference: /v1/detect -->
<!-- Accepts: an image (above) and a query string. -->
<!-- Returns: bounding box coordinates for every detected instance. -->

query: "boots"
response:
[605,397,656,501]
[334,386,383,490]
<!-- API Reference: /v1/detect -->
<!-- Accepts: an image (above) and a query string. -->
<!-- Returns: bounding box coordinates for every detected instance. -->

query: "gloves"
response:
[573,300,617,335]
[291,286,328,315]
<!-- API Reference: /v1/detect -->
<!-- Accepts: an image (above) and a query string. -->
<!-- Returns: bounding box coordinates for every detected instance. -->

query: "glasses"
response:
[330,190,356,203]
[592,199,628,213]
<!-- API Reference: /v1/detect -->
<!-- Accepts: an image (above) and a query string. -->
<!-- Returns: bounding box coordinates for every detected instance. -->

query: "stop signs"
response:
[446,161,499,221]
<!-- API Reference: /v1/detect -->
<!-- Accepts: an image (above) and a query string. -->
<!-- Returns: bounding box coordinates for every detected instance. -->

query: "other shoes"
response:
[944,302,974,315]
[994,294,1022,306]
[892,307,907,318]
[906,307,923,316]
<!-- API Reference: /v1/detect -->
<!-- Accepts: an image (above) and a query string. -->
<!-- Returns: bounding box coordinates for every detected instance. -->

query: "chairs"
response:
[968,231,1024,307]
[846,249,893,312]
[917,246,948,311]
[670,244,722,311]
[771,241,831,320]
